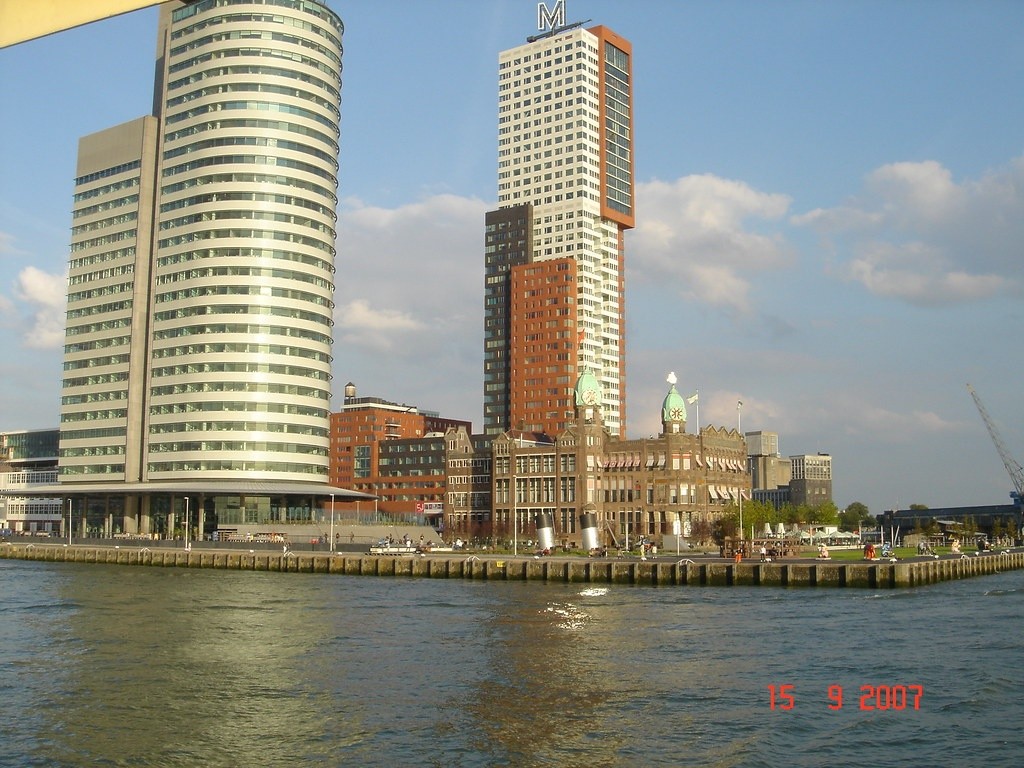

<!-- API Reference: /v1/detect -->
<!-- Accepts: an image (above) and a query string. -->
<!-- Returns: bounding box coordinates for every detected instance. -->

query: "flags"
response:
[578,331,584,342]
[687,393,698,404]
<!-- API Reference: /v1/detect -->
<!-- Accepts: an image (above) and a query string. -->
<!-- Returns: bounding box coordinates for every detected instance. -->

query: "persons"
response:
[283,541,290,554]
[452,538,465,550]
[652,544,657,558]
[528,538,532,547]
[385,533,432,553]
[247,532,250,539]
[349,532,354,544]
[719,546,723,557]
[736,546,741,562]
[589,548,605,558]
[918,542,937,555]
[318,532,328,543]
[540,548,549,555]
[760,546,766,562]
[336,532,339,541]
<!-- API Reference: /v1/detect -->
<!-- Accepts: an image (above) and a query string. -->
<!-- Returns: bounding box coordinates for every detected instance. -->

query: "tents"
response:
[786,529,860,545]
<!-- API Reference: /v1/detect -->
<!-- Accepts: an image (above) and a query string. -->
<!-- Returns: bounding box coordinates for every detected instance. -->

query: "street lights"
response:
[329,494,334,551]
[184,497,189,549]
[740,490,745,552]
[512,475,517,558]
[68,499,72,545]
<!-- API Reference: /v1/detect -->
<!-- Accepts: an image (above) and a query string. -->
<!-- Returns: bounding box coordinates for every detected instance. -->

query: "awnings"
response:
[696,460,743,470]
[597,459,665,468]
[709,490,748,499]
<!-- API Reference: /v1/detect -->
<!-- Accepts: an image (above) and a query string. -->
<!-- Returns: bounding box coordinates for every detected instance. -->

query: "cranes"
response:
[966,383,1024,545]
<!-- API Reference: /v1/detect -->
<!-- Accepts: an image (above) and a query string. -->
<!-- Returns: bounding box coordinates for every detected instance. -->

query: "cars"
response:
[36,530,54,538]
[0,528,12,537]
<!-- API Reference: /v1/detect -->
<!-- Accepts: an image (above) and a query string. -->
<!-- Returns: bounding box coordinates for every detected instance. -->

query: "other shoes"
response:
[760,560,762,563]
[763,559,765,562]
